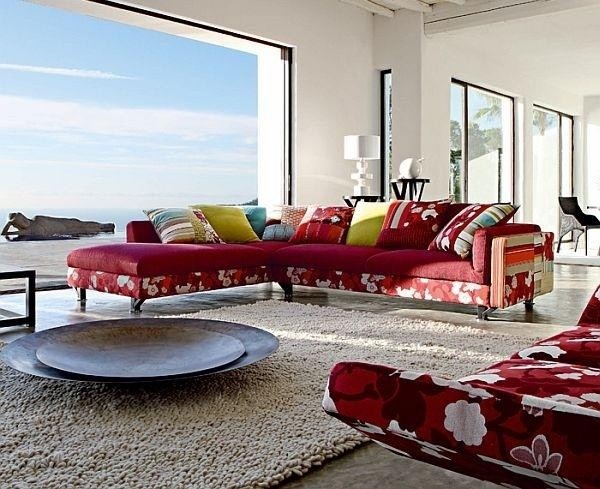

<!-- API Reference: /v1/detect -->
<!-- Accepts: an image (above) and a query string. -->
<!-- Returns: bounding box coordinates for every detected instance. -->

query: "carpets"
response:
[0,299,546,489]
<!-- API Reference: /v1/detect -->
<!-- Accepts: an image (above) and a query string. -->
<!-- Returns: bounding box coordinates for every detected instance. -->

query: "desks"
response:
[0,264,36,332]
[390,178,431,203]
[342,196,379,209]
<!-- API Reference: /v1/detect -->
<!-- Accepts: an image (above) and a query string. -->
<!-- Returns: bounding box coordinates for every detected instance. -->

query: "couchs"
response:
[67,220,554,321]
[321,285,599,489]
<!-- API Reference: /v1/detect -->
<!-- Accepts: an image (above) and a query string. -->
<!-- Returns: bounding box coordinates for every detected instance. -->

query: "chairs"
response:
[557,197,600,256]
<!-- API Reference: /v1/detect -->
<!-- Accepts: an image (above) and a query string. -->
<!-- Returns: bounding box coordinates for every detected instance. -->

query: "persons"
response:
[1,212,115,237]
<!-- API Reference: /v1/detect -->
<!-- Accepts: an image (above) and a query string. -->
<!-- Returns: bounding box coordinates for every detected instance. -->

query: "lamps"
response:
[343,135,381,196]
